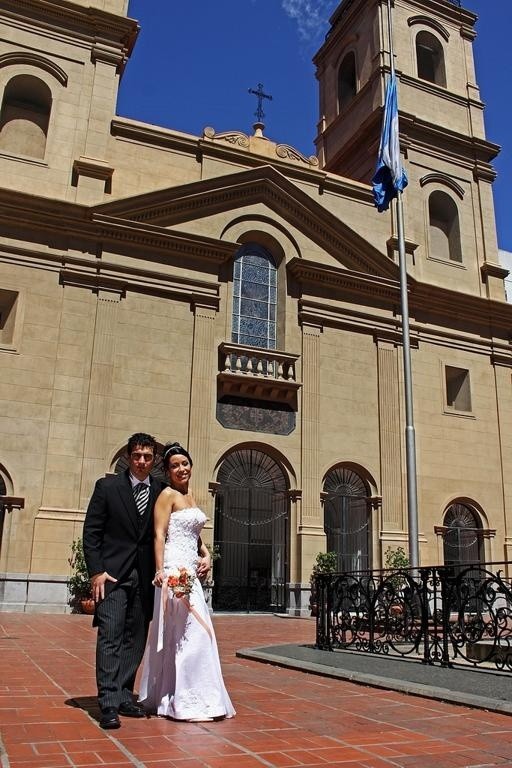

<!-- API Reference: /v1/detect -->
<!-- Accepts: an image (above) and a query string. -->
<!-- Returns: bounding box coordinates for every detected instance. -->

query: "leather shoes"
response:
[98,708,123,729]
[120,700,146,718]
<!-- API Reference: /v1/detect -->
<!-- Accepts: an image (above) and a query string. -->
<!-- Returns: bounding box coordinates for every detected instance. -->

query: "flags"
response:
[370,71,408,213]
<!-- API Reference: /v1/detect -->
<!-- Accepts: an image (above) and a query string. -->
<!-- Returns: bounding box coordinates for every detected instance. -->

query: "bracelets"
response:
[155,571,162,577]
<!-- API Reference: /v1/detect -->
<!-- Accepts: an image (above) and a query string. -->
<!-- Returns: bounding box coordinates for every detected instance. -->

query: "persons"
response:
[138,442,238,723]
[82,432,212,728]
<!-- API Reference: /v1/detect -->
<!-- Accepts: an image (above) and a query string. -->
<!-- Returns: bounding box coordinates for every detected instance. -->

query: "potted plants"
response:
[67,538,96,614]
[311,546,411,615]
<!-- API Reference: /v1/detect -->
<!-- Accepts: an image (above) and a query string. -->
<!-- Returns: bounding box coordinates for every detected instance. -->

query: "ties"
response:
[135,483,149,515]
